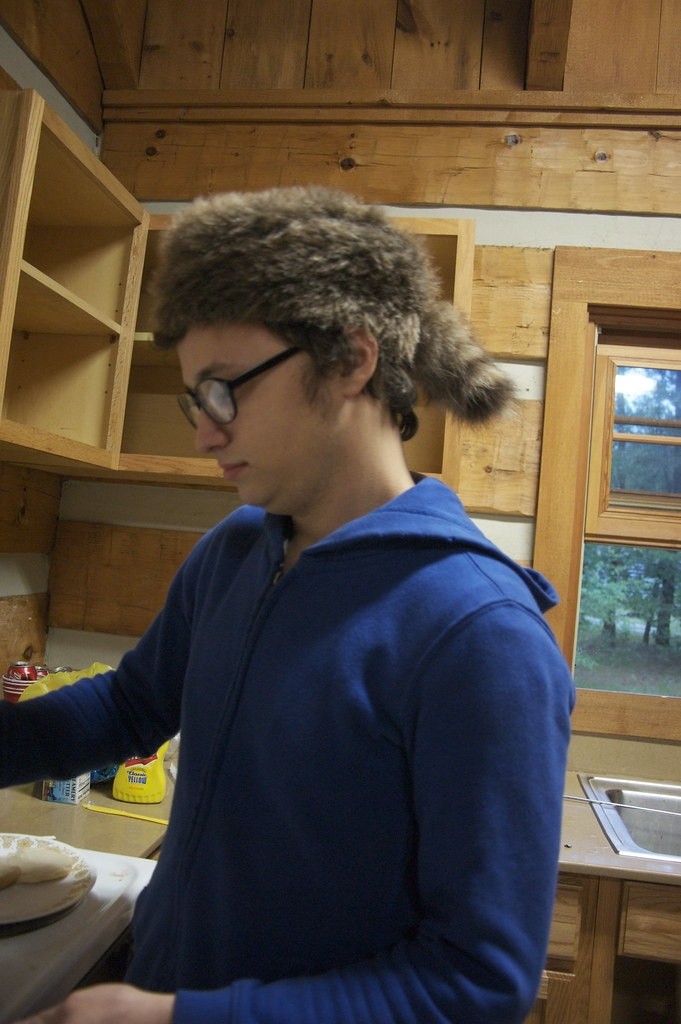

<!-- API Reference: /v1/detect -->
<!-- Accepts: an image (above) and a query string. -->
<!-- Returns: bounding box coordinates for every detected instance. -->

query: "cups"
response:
[3,673,38,703]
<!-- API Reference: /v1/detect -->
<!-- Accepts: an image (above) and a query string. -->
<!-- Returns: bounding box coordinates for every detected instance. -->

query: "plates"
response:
[0,833,96,924]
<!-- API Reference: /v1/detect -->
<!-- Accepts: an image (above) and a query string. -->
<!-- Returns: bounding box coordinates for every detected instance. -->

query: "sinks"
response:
[576,773,681,864]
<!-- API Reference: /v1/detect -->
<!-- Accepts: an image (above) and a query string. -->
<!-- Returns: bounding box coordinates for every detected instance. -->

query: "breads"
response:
[0,856,21,890]
[8,847,71,884]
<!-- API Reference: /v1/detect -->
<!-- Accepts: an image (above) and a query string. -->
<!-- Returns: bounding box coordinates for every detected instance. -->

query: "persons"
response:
[0,184,577,1023]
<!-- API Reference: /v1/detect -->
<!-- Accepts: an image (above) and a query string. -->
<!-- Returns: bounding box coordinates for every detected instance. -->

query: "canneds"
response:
[6,660,72,681]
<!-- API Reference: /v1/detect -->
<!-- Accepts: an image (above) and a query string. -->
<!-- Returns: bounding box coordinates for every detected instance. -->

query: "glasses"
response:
[173,342,302,430]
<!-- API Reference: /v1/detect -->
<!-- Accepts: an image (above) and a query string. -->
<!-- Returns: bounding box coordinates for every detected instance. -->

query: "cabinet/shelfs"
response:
[78,215,477,493]
[0,89,150,472]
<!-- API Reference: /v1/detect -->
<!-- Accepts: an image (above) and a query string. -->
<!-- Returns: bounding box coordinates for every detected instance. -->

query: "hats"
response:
[156,184,514,422]
